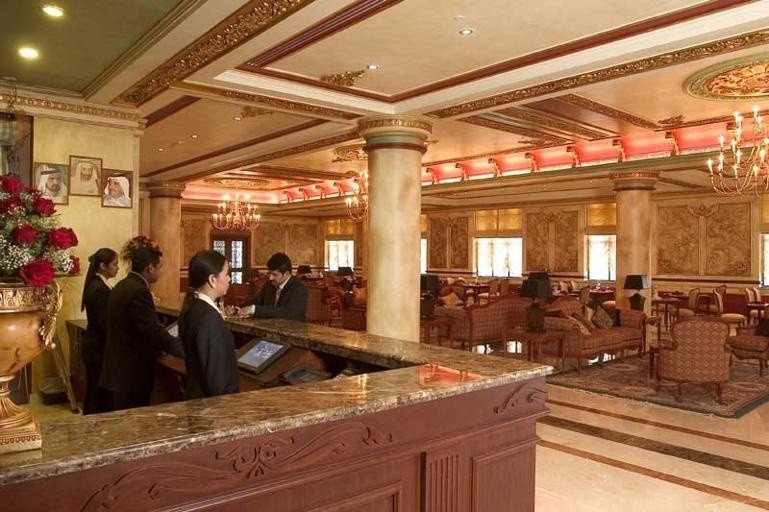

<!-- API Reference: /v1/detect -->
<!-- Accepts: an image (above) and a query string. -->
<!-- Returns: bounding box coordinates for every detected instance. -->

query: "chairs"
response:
[228,274,365,330]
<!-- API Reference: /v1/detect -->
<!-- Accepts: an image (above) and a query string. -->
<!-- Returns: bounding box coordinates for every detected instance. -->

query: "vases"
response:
[1,281,65,459]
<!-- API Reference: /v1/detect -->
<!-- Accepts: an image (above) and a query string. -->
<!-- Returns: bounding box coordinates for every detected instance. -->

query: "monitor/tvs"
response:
[236,337,293,375]
[163,321,178,339]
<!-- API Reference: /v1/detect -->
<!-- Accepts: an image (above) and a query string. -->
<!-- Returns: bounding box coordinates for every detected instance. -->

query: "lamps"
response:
[706,100,767,203]
[209,174,261,234]
[342,169,368,223]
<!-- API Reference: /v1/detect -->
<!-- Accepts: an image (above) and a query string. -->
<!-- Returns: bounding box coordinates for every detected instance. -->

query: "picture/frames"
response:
[34,163,69,206]
[0,111,33,192]
[68,154,101,197]
[100,167,133,210]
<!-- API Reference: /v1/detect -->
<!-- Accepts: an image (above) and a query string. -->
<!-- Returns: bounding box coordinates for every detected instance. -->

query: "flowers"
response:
[1,174,85,292]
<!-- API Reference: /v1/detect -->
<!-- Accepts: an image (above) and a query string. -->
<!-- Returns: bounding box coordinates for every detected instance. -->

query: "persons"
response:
[71,159,100,195]
[99,244,185,412]
[179,251,238,399]
[102,173,133,205]
[39,167,69,204]
[82,247,119,417]
[227,251,312,323]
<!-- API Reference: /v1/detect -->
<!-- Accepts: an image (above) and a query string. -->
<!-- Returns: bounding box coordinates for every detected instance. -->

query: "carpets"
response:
[543,348,768,420]
